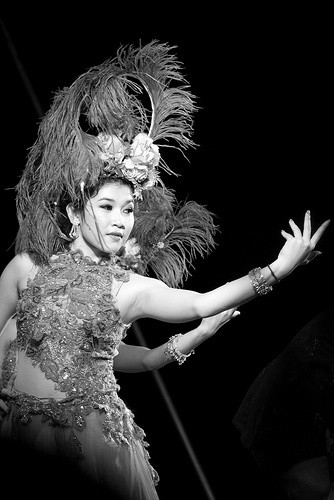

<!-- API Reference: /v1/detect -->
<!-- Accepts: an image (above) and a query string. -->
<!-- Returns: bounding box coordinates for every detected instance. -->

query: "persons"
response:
[1,182,241,388]
[1,39,330,500]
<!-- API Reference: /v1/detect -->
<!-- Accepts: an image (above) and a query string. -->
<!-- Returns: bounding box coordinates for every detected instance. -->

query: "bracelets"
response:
[165,333,196,366]
[247,265,282,296]
